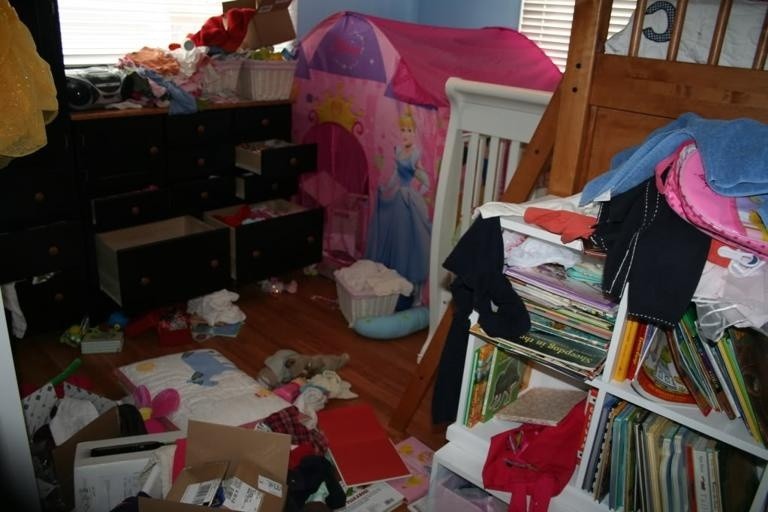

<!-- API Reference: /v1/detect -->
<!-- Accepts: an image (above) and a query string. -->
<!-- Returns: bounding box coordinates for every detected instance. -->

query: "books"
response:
[460,259,767,512]
[81,332,125,355]
[318,402,437,512]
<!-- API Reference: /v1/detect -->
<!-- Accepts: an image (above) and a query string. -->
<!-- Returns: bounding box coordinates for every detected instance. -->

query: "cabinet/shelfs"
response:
[0,97,327,346]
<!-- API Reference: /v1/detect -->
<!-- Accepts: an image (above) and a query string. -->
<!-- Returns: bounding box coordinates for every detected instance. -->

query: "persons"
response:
[362,104,433,306]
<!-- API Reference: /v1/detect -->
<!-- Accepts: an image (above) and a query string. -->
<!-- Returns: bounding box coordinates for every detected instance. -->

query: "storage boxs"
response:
[72,418,293,512]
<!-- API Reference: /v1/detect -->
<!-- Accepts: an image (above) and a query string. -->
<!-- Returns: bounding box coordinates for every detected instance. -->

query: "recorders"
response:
[65,66,134,111]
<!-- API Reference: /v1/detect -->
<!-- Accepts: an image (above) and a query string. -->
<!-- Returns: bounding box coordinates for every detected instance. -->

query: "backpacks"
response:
[654,138,768,262]
